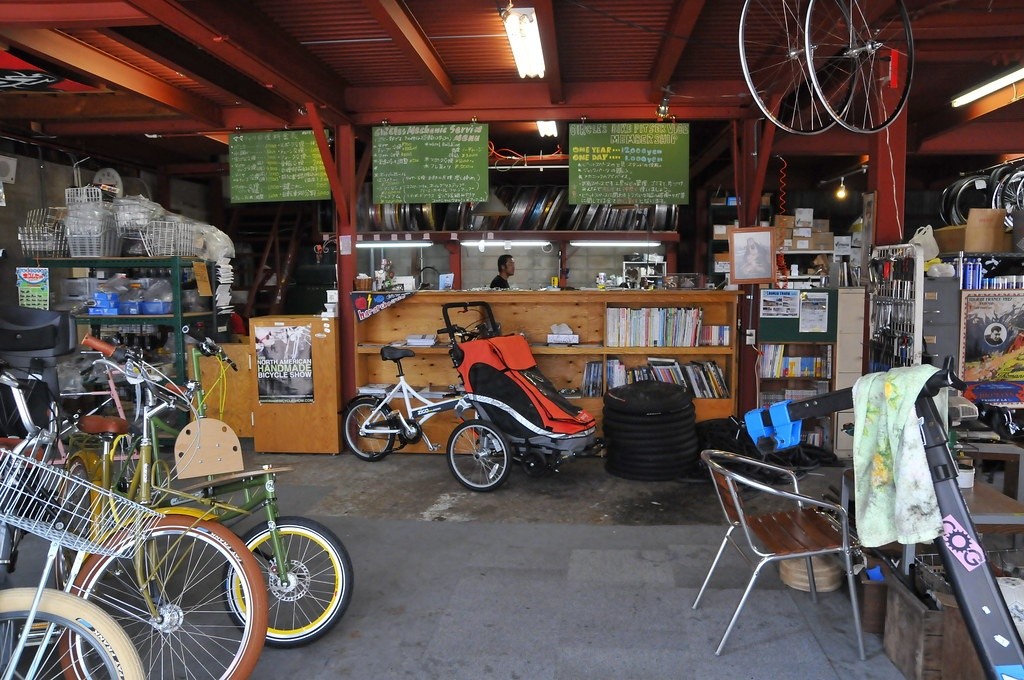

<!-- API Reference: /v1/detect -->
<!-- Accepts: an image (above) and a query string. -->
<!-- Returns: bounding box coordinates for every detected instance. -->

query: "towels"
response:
[851,363,949,548]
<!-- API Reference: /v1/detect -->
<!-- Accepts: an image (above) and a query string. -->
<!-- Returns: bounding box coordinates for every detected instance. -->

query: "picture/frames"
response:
[728,226,778,285]
[958,289,1024,409]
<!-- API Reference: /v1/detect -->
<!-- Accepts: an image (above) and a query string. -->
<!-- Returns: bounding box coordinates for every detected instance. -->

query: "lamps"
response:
[501,5,547,79]
[837,175,848,200]
[569,239,663,248]
[460,239,550,247]
[949,62,1024,109]
[538,119,558,141]
[356,242,434,248]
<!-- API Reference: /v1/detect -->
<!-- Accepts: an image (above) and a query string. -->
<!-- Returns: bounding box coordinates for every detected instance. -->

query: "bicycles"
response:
[338,324,512,492]
[0,334,269,680]
[55,324,354,651]
[0,358,164,680]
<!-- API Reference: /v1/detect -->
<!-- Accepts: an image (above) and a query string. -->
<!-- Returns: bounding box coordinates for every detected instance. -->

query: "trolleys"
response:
[442,301,607,478]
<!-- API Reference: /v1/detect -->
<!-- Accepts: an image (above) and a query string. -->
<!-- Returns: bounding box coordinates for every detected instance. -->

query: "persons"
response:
[985,326,1005,345]
[490,254,515,290]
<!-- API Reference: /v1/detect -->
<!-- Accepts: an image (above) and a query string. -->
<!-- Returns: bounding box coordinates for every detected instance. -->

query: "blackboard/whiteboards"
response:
[230,128,331,205]
[568,123,690,204]
[371,123,488,204]
[758,288,838,345]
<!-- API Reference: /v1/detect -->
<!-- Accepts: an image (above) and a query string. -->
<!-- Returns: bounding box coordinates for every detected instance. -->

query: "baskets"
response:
[18,187,196,256]
[0,447,166,559]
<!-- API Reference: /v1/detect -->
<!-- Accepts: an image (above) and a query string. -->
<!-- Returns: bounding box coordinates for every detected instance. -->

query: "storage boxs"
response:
[774,228,792,246]
[812,282,820,287]
[795,207,814,228]
[812,232,834,250]
[834,232,862,267]
[779,282,793,289]
[714,251,730,273]
[883,576,943,680]
[794,282,812,289]
[792,237,813,250]
[775,215,794,228]
[711,225,734,240]
[859,559,891,633]
[792,227,811,238]
[811,219,829,232]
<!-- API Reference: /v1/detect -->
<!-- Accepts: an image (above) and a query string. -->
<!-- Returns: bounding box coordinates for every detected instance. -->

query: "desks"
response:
[954,438,1024,498]
[840,466,1024,574]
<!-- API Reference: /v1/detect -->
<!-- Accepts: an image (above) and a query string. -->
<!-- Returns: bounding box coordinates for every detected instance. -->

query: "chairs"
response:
[694,448,866,659]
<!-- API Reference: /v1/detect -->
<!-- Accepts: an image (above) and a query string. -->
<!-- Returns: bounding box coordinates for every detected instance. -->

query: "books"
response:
[581,307,730,399]
[356,334,465,399]
[759,345,832,448]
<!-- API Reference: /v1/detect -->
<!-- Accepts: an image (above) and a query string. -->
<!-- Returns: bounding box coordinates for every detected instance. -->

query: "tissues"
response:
[547,323,580,344]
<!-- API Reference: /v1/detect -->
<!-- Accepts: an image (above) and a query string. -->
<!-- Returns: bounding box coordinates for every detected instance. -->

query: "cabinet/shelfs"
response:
[623,260,668,290]
[758,341,835,453]
[189,336,254,441]
[32,255,216,447]
[774,249,835,288]
[352,290,739,454]
[251,313,343,458]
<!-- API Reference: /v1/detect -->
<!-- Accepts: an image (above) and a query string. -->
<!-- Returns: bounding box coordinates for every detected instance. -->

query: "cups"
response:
[551,277,558,288]
[981,277,995,289]
[996,276,1016,290]
[1017,276,1024,289]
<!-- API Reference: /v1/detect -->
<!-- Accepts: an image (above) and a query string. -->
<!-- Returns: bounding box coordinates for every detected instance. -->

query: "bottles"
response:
[627,277,637,288]
[641,280,655,290]
[596,273,623,289]
[943,258,960,278]
[963,258,982,290]
